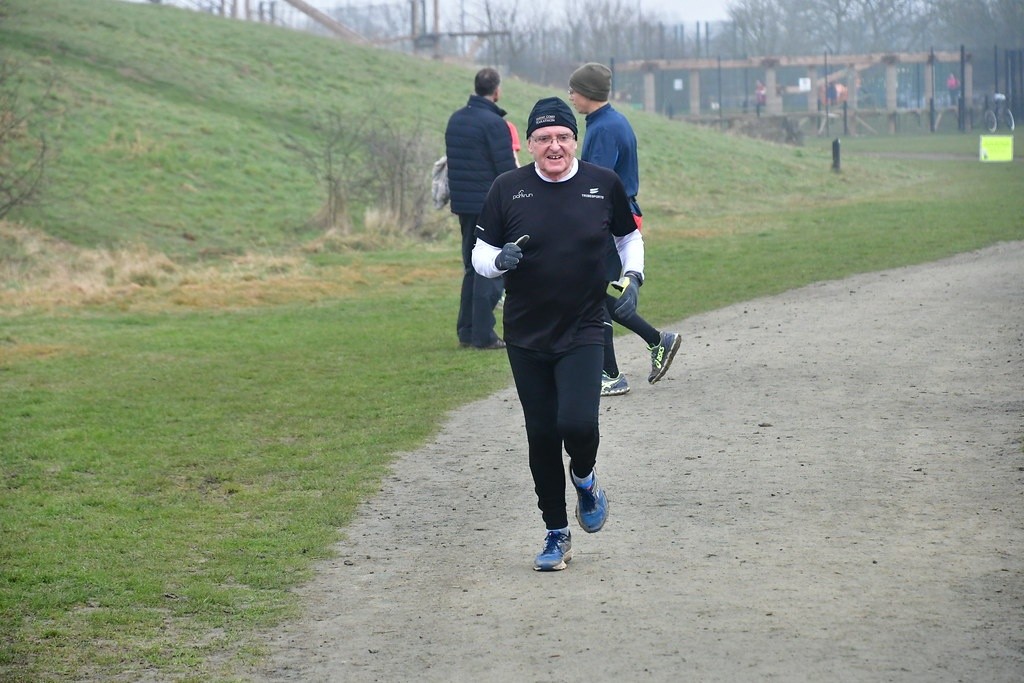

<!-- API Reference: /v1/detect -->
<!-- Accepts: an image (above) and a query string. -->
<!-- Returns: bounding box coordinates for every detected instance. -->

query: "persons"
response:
[945,73,960,108]
[567,62,683,396]
[471,97,646,571]
[445,67,508,351]
[503,118,522,169]
[753,80,766,118]
[827,83,838,109]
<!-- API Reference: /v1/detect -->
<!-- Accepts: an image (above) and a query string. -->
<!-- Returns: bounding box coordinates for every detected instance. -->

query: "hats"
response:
[570,62,612,102]
[526,97,578,141]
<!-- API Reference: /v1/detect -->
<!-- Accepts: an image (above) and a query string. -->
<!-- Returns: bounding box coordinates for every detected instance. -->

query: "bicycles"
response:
[984,99,1015,133]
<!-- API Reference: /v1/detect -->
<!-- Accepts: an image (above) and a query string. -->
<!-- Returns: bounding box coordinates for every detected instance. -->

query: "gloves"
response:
[495,235,530,271]
[611,270,643,321]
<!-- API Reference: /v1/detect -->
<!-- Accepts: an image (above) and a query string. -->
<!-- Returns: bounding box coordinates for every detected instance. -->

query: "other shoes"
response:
[471,337,507,350]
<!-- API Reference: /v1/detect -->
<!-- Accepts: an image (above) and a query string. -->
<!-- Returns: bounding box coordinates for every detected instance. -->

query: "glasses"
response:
[530,135,576,145]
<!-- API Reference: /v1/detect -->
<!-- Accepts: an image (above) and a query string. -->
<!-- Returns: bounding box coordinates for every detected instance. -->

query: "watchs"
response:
[624,270,643,287]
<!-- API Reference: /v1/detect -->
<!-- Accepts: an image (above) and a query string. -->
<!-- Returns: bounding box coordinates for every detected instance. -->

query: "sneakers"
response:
[532,530,573,572]
[646,331,681,384]
[599,369,630,395]
[568,461,608,533]
